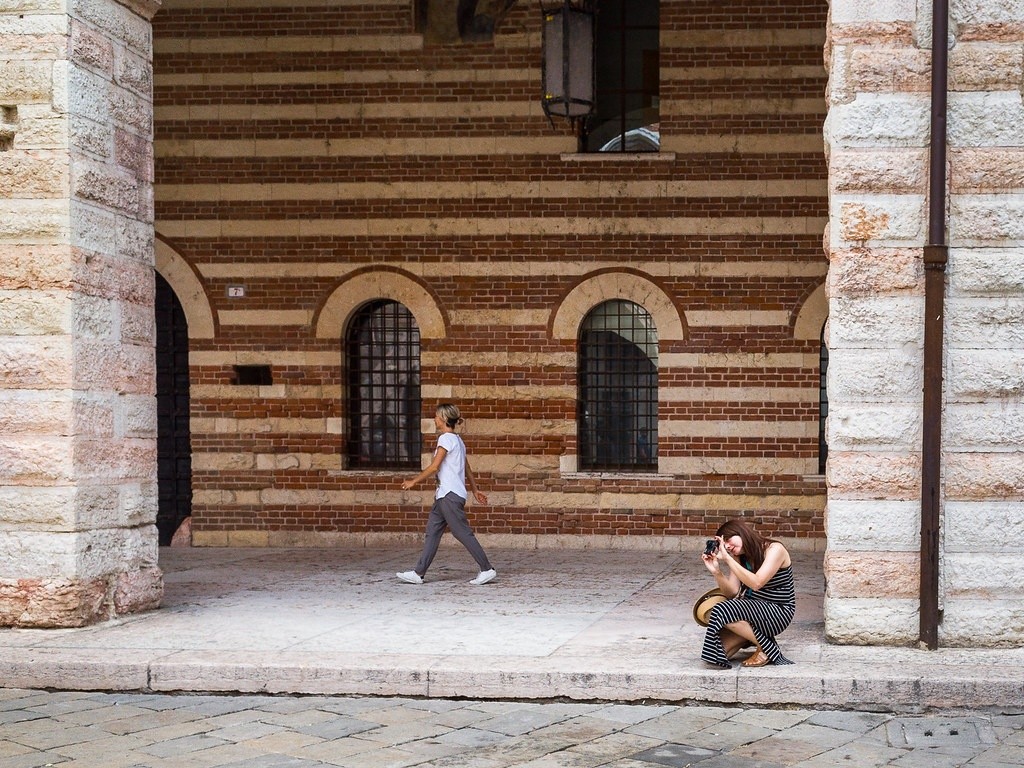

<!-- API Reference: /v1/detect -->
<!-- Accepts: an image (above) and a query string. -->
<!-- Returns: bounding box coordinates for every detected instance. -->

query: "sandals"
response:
[742,646,770,667]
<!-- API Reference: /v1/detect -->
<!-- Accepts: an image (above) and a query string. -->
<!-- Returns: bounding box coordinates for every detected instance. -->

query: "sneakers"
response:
[396,571,424,584]
[469,567,496,585]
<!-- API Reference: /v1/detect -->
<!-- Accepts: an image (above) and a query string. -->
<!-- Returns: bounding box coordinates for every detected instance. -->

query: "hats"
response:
[693,588,729,627]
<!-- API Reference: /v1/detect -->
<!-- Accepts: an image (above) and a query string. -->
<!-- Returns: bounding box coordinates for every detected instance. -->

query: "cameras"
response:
[704,540,719,556]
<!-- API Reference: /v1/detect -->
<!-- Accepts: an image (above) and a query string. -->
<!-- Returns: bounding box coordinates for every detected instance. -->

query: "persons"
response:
[396,403,496,585]
[637,427,650,463]
[594,420,616,464]
[354,426,394,463]
[701,519,796,669]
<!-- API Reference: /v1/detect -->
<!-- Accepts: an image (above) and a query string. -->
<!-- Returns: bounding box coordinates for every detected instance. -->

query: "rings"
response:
[402,487,405,489]
